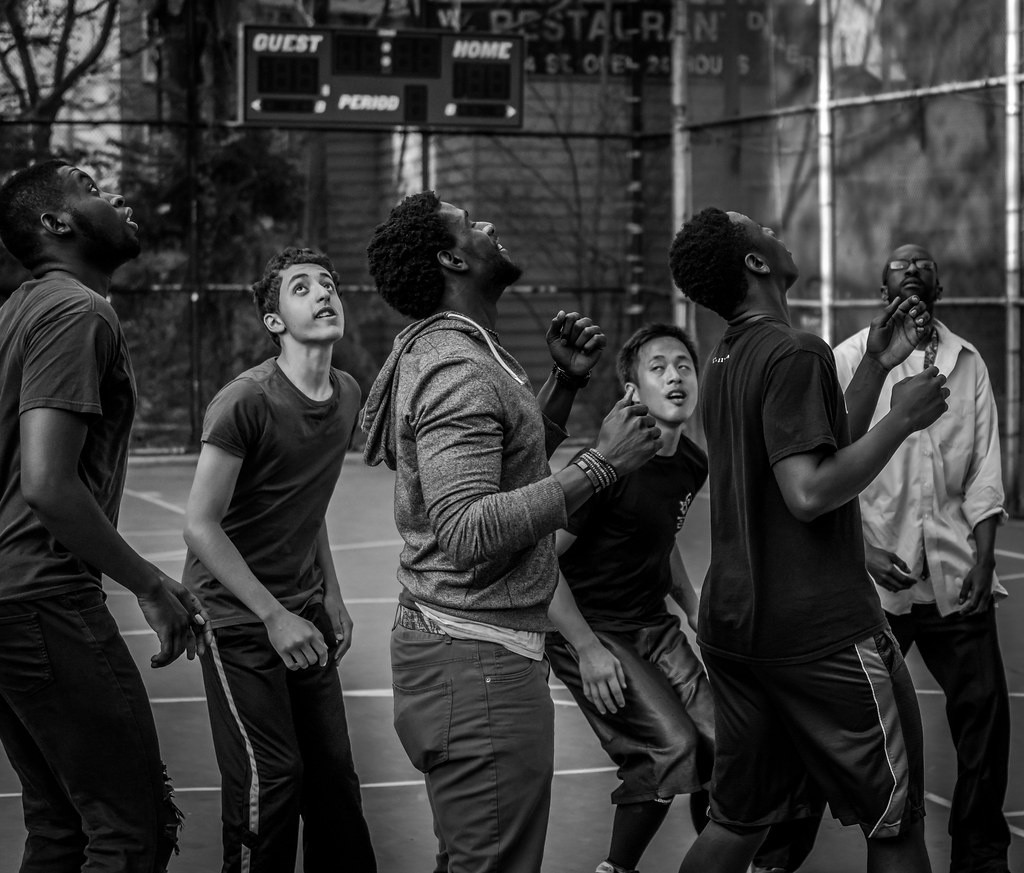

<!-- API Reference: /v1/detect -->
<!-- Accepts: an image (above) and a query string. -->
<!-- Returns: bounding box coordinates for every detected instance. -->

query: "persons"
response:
[0,160,216,872]
[358,192,663,873]
[669,208,949,872]
[545,322,716,873]
[831,244,1012,873]
[181,245,377,873]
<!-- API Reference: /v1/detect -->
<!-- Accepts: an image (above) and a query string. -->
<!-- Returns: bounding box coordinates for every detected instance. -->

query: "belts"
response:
[398,604,447,635]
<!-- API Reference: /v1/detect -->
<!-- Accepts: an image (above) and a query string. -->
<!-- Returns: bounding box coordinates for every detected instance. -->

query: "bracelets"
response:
[552,363,592,387]
[575,448,618,493]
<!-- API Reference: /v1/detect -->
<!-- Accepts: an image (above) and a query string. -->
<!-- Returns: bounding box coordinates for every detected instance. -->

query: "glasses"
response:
[882,257,938,287]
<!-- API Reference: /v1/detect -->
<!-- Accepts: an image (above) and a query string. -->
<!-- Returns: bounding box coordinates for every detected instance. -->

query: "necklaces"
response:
[484,327,499,337]
